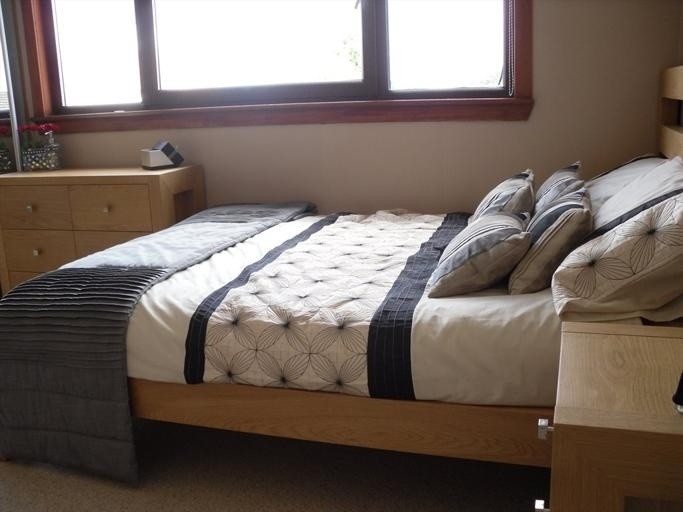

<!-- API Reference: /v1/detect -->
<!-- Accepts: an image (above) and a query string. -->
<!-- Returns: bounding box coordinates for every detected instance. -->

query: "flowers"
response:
[0,124,12,137]
[18,121,61,137]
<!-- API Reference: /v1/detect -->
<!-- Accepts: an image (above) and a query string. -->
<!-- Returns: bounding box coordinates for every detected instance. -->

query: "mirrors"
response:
[0,6,21,174]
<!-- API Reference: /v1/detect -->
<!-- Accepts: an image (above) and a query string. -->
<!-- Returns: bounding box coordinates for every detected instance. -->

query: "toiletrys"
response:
[44,129,62,169]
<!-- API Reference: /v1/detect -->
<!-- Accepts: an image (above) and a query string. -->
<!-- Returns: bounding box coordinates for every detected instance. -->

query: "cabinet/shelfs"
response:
[0,164,205,304]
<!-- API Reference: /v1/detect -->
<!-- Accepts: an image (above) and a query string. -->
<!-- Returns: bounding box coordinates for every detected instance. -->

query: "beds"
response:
[0,63,683,469]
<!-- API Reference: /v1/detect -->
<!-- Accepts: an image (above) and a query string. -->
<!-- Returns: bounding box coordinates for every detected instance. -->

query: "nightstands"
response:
[545,319,683,511]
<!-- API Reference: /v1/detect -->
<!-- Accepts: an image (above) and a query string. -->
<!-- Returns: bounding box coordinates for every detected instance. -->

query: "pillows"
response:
[427,210,534,299]
[534,159,585,216]
[550,155,682,318]
[508,185,595,297]
[467,166,538,227]
